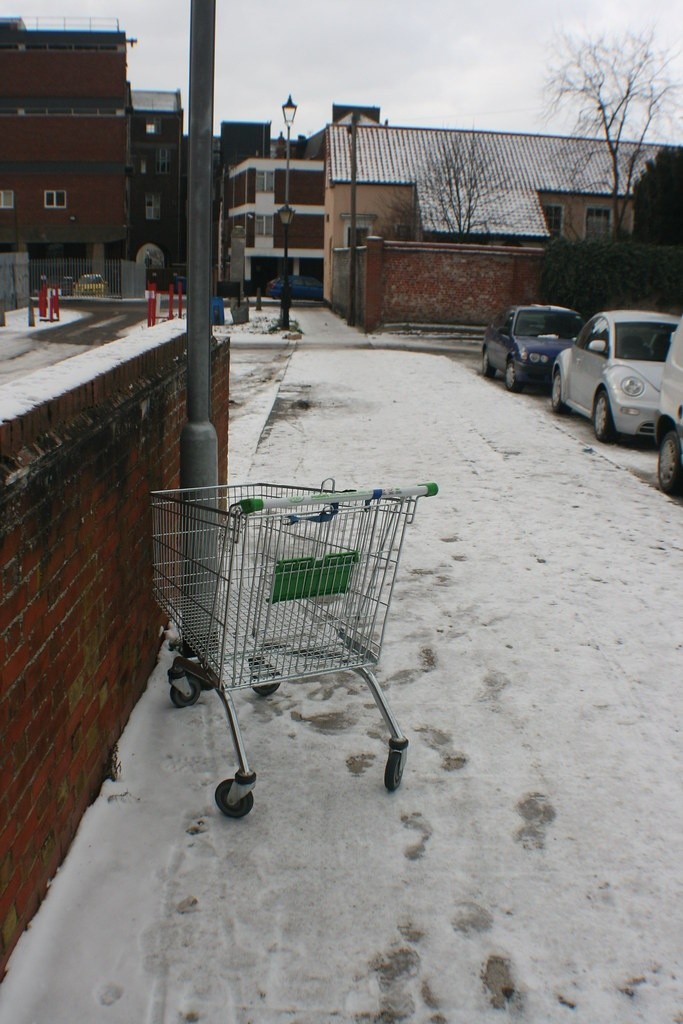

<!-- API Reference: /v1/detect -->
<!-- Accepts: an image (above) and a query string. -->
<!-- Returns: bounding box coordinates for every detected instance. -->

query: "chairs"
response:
[646,334,670,360]
[619,335,652,362]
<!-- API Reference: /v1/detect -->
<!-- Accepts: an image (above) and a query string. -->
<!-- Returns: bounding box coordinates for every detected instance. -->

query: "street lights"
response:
[281,94,299,206]
[277,203,295,327]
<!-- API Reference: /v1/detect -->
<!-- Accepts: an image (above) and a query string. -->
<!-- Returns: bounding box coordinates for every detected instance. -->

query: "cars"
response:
[46,274,75,296]
[481,302,587,394]
[266,274,324,301]
[72,274,109,296]
[654,310,683,496]
[551,310,683,444]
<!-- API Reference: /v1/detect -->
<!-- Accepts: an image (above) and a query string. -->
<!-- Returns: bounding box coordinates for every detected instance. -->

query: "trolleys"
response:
[151,477,439,821]
